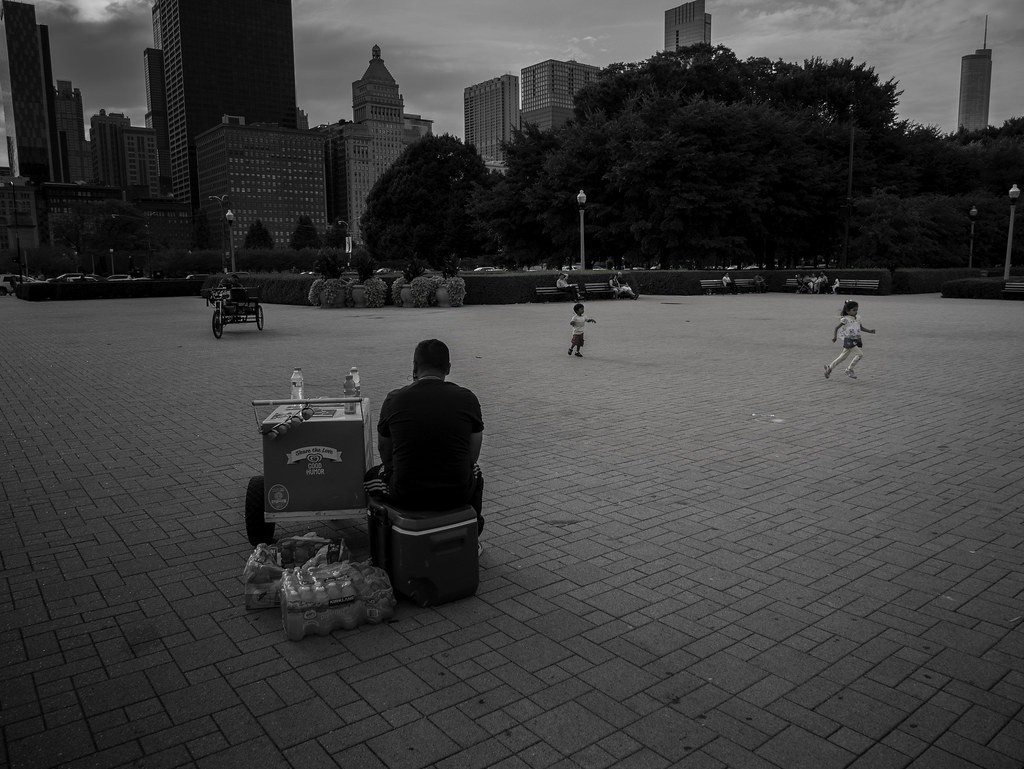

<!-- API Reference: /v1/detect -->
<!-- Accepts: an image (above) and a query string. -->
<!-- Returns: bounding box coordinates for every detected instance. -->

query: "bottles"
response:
[243,532,397,641]
[291,367,305,400]
[343,375,357,414]
[350,367,361,397]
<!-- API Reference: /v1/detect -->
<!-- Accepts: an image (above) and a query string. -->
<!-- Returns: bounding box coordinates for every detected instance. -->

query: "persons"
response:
[753,275,767,293]
[568,304,596,357]
[832,273,840,294]
[609,273,622,300]
[555,273,584,302]
[8,276,17,296]
[722,273,741,294]
[363,338,485,556]
[807,273,817,294]
[823,301,876,379]
[814,271,828,293]
[617,272,640,300]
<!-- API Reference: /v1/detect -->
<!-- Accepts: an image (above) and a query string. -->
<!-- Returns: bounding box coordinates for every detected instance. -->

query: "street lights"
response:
[225,210,236,272]
[209,194,230,273]
[338,217,360,271]
[577,189,586,269]
[109,248,114,274]
[968,205,978,268]
[1004,183,1020,282]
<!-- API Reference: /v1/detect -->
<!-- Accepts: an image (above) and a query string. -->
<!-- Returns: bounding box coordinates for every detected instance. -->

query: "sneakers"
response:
[844,367,857,378]
[824,364,832,378]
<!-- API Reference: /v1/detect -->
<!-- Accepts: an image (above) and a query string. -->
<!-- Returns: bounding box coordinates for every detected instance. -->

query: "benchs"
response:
[700,279,756,294]
[783,278,879,295]
[584,283,625,301]
[1001,281,1024,300]
[536,284,581,304]
[208,285,263,303]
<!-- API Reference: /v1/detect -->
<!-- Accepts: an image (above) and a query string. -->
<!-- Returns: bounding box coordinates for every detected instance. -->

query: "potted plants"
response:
[308,244,467,309]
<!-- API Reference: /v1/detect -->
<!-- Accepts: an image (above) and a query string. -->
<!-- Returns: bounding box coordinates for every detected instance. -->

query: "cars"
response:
[0,272,209,297]
[375,259,841,274]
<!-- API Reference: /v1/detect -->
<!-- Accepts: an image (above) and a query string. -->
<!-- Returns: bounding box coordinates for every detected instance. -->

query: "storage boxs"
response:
[260,397,371,514]
[367,496,479,609]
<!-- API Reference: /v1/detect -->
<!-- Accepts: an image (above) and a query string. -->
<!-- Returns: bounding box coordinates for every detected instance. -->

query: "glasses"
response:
[291,408,314,429]
[268,424,289,440]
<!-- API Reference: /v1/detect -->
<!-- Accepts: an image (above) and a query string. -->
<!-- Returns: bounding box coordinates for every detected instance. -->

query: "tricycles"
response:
[206,285,263,340]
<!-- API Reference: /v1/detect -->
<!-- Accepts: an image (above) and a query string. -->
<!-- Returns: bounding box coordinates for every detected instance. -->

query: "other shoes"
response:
[634,293,639,300]
[578,296,584,299]
[568,348,573,355]
[575,352,582,357]
